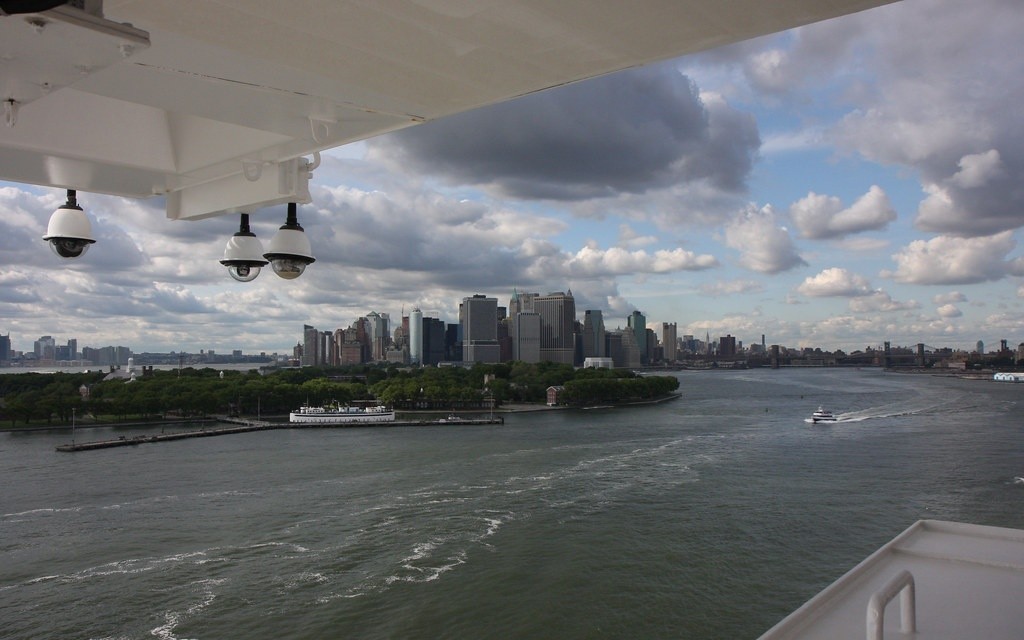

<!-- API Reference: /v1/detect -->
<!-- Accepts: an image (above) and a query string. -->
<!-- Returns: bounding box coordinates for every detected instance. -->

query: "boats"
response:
[812,404,837,421]
[289,395,396,423]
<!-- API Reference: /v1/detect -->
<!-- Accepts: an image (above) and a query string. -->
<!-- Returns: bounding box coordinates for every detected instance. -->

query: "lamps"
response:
[264,201,317,279]
[42,190,96,261]
[220,214,269,282]
